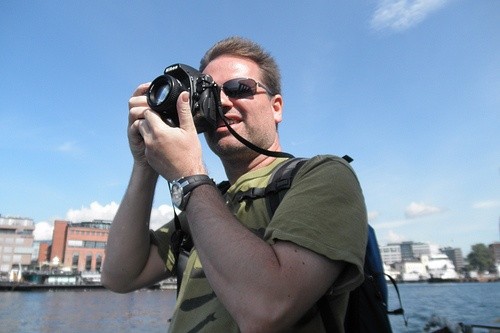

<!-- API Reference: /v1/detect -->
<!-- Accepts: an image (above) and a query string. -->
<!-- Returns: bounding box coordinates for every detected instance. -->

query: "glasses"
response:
[216,77,275,99]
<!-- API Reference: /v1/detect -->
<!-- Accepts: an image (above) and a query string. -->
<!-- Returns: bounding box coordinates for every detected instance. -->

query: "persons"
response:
[101,35,368,333]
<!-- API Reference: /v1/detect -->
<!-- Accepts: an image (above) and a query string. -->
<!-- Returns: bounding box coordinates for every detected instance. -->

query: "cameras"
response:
[147,63,219,134]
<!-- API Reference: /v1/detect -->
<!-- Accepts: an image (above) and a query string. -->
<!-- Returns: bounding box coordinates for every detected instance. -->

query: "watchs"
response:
[171,174,216,210]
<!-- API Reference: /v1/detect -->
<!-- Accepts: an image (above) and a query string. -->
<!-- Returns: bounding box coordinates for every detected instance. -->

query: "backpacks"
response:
[175,151,394,333]
